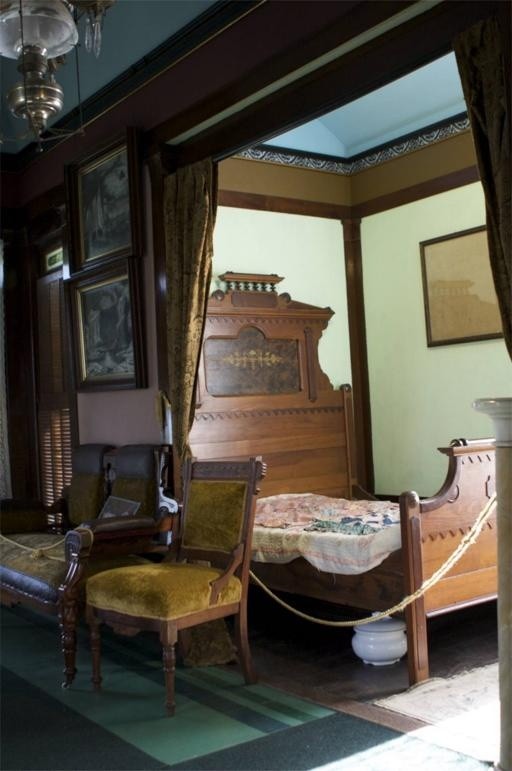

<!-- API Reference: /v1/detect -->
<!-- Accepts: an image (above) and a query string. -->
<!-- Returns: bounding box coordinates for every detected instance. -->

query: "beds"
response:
[190,268,496,691]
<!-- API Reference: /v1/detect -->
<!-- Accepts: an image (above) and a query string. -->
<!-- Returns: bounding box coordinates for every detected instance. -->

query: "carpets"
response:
[0,602,435,768]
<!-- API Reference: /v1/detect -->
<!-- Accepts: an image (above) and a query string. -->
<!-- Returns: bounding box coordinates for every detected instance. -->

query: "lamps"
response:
[0,0,113,151]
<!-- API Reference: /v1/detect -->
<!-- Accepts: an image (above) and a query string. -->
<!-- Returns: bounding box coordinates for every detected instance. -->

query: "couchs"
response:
[0,438,174,688]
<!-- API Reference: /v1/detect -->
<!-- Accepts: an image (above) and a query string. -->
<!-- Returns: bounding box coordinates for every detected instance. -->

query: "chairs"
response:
[89,457,262,718]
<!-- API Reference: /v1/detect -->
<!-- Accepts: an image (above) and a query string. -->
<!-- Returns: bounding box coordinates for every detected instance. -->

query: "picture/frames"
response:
[60,130,152,392]
[417,222,505,347]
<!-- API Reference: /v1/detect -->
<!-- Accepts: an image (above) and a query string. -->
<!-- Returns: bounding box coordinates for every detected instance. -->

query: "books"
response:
[98,496,141,521]
